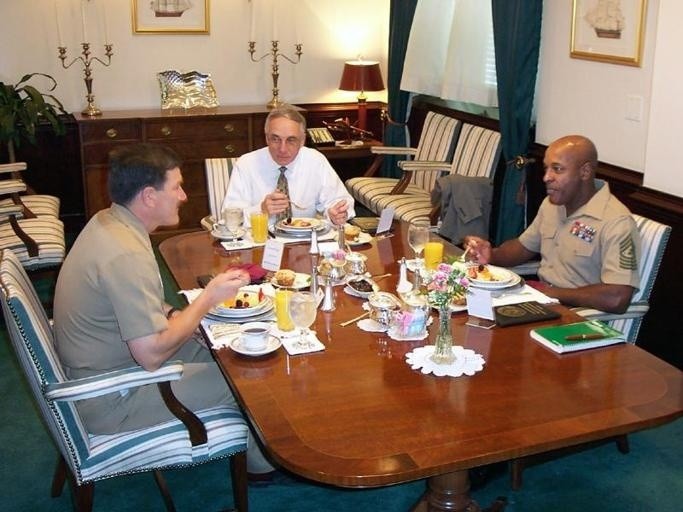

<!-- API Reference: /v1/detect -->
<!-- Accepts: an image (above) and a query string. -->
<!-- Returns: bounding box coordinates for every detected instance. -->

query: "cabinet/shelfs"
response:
[80,118,144,222]
[251,110,277,155]
[154,114,250,221]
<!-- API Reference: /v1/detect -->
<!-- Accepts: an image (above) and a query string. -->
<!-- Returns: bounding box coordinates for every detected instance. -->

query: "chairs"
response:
[340,109,462,220]
[375,117,499,231]
[1,248,255,512]
[499,209,674,347]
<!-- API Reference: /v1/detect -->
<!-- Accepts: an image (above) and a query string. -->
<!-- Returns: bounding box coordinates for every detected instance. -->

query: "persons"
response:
[50,138,280,492]
[461,135,644,317]
[218,101,359,232]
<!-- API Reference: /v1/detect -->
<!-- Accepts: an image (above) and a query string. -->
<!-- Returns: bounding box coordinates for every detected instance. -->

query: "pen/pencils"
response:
[566,334,604,339]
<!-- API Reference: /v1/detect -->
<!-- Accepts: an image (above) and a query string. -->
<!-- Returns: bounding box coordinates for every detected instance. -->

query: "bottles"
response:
[315,254,367,282]
[412,269,420,292]
[396,257,410,291]
[337,225,344,249]
[367,290,431,340]
[308,266,318,293]
[307,226,318,256]
[319,278,334,313]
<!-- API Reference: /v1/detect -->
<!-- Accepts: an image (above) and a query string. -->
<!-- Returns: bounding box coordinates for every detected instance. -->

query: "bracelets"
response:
[166,307,181,320]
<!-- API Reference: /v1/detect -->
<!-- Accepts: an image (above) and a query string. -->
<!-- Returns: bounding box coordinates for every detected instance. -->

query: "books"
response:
[195,265,271,287]
[528,318,627,355]
[348,214,396,234]
[491,299,562,327]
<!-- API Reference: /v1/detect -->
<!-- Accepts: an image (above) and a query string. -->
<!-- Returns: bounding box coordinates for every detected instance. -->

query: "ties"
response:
[275,166,293,222]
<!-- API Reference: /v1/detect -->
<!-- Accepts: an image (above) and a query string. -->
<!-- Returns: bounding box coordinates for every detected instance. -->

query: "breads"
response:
[276,268,295,285]
[343,226,361,242]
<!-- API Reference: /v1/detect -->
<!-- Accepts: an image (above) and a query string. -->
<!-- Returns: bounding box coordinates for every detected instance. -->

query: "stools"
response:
[0,184,69,314]
[0,158,63,222]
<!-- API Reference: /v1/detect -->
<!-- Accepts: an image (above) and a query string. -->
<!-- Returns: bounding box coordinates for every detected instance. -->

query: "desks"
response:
[300,134,387,181]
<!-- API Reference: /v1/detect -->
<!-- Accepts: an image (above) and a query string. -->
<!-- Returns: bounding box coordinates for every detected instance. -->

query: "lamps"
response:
[336,53,385,139]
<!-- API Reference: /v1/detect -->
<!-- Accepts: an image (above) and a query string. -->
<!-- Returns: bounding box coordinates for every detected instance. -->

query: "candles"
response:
[76,0,89,43]
[100,4,113,45]
[272,0,282,40]
[293,0,301,43]
[54,0,63,46]
[248,0,256,45]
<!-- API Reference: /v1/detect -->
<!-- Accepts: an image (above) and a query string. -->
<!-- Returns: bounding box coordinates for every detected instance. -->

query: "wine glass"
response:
[291,292,317,353]
[403,223,430,294]
[224,208,246,248]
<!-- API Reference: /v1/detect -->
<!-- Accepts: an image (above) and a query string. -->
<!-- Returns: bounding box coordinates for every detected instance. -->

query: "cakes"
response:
[473,264,492,280]
[294,218,315,228]
[223,293,259,307]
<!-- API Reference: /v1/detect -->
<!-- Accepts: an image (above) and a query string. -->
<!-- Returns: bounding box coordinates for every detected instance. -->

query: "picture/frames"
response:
[568,1,648,69]
[131,0,211,36]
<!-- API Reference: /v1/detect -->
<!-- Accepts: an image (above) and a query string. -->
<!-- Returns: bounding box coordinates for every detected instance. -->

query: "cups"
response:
[274,286,297,335]
[425,224,444,273]
[241,322,270,349]
[251,209,271,246]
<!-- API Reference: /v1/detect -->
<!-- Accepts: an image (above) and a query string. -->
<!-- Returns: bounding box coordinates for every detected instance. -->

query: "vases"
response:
[432,305,454,364]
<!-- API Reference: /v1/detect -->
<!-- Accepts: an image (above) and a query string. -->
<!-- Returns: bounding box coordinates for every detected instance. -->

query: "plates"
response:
[230,335,284,355]
[343,275,379,297]
[271,217,327,234]
[452,261,523,291]
[335,232,371,246]
[207,290,272,319]
[271,273,312,290]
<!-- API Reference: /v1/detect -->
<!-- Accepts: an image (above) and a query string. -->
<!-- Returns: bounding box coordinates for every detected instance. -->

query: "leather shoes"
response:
[245,467,314,489]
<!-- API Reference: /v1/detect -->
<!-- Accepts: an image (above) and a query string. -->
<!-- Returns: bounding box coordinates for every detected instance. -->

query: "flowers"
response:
[413,259,471,310]
